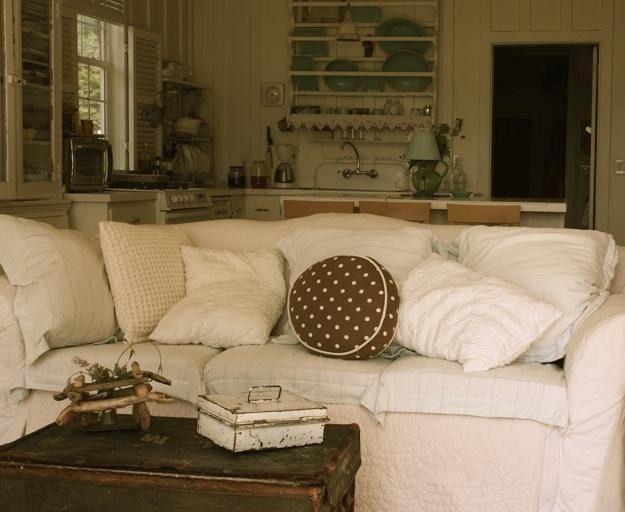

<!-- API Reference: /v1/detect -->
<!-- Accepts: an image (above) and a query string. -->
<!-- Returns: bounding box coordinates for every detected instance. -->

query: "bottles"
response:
[227,166,246,188]
[250,160,268,188]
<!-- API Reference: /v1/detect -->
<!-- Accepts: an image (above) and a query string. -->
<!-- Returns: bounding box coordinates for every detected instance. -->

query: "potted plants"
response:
[407,118,463,198]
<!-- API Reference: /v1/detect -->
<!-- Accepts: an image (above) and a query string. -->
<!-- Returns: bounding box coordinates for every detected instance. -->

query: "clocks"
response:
[262,83,284,107]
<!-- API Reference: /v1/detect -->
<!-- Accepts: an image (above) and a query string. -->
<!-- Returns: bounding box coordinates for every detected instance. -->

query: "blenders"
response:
[272,144,297,189]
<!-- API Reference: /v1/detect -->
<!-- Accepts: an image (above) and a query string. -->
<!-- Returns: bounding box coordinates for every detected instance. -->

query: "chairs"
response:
[283,200,521,227]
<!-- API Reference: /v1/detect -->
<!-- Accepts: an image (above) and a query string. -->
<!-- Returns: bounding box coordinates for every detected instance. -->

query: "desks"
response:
[0,414,362,512]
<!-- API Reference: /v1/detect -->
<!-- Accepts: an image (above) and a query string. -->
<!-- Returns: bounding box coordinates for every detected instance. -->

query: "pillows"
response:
[149,246,285,353]
[277,217,440,261]
[394,253,561,372]
[289,256,401,360]
[3,214,119,364]
[460,227,616,363]
[98,219,192,344]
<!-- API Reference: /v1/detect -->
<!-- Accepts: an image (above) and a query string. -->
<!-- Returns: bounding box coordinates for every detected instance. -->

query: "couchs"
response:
[0,213,625,512]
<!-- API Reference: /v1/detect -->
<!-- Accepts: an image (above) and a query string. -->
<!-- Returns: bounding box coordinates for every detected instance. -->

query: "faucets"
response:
[341,141,360,170]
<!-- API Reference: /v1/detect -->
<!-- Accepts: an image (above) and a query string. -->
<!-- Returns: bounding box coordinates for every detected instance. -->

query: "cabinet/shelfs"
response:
[161,79,211,143]
[69,201,155,233]
[212,197,245,218]
[0,0,63,200]
[244,195,280,221]
[287,0,441,130]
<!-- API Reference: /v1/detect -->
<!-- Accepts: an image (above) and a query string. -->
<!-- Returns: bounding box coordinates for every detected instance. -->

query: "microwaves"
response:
[62,135,114,193]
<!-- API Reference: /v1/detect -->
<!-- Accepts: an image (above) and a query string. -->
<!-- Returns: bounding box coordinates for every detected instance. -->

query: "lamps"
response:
[406,133,442,199]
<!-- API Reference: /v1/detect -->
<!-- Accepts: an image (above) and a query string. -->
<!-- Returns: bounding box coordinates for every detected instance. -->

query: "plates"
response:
[375,18,433,57]
[323,59,360,92]
[350,7,383,23]
[450,191,473,199]
[290,53,320,93]
[383,51,431,92]
[292,26,330,58]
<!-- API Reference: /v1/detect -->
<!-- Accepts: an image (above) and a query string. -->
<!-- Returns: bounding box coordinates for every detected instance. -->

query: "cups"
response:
[362,41,374,57]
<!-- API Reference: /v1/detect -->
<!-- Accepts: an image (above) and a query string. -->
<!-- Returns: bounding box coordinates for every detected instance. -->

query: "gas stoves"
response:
[105,181,213,212]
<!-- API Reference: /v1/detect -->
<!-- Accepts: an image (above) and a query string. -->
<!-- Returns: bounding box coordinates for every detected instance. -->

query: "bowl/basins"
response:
[381,98,405,116]
